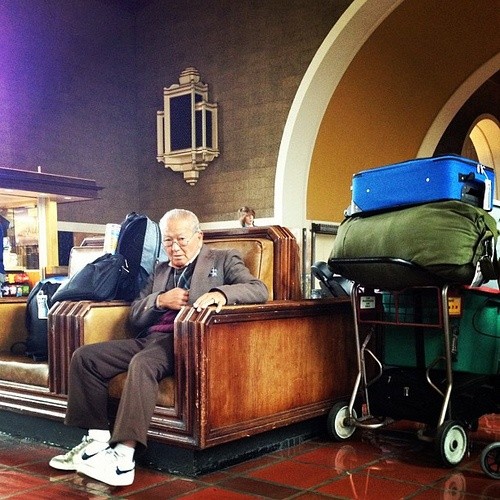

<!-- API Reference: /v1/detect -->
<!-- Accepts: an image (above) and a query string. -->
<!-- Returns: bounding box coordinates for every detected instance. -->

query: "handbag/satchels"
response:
[50,252,129,301]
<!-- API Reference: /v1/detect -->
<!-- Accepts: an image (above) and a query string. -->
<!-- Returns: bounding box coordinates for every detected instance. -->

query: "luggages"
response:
[343,153,495,217]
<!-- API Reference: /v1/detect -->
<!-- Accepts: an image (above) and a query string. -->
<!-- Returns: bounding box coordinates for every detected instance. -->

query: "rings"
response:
[211,298,215,303]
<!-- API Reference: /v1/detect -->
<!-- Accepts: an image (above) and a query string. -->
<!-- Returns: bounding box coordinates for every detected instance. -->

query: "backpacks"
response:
[114,211,161,302]
[24,281,61,362]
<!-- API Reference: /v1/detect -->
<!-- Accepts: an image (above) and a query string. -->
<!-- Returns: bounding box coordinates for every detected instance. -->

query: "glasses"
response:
[162,231,199,247]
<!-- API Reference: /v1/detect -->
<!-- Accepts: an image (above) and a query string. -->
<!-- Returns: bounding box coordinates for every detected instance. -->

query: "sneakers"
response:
[48,435,111,470]
[77,447,136,486]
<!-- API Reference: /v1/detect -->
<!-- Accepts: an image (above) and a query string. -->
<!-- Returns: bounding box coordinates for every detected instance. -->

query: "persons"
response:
[49,208,269,486]
[237,206,256,228]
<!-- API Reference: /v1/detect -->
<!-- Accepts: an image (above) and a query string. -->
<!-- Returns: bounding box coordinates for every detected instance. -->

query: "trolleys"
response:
[312,237,500,466]
[329,438,467,500]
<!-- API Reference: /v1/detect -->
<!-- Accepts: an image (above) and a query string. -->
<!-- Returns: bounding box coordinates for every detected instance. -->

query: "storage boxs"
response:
[379,290,500,376]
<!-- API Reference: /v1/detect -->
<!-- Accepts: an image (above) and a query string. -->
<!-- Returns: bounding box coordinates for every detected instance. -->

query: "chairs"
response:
[0,224,355,449]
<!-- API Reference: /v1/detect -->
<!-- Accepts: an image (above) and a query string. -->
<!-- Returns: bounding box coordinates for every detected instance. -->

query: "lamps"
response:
[158,67,218,186]
